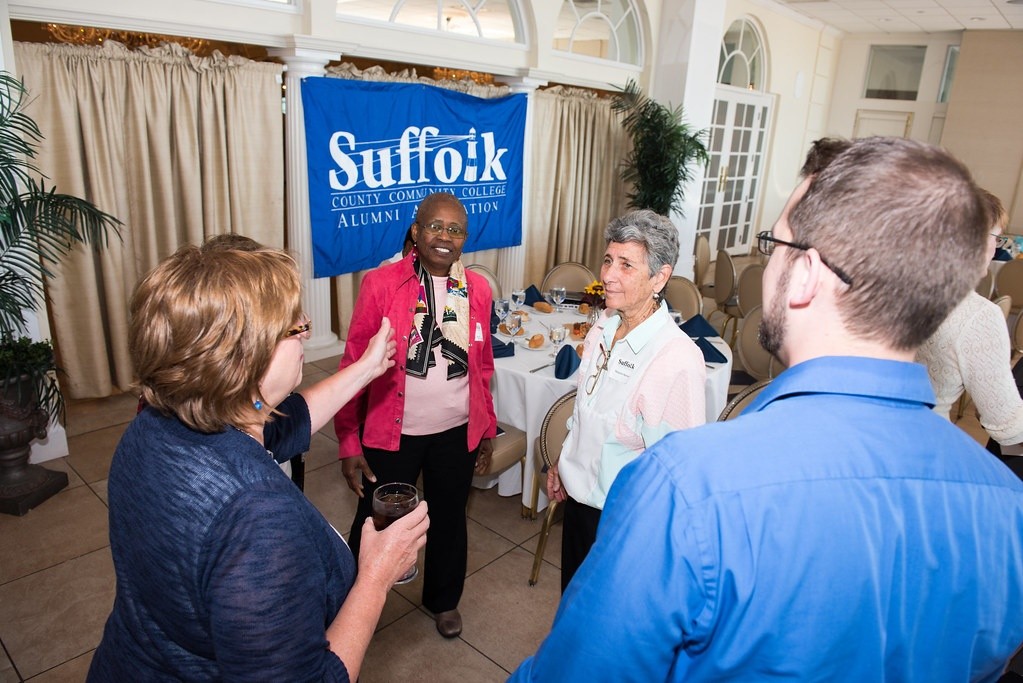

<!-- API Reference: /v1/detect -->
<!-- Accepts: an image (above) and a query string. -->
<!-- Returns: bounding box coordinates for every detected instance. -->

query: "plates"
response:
[573,309,588,318]
[497,329,526,338]
[532,308,549,317]
[519,338,543,352]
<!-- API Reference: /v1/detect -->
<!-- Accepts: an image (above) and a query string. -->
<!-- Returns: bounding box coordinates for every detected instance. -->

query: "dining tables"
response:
[480,309,601,518]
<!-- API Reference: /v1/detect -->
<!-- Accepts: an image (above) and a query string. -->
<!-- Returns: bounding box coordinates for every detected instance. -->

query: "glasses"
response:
[585,342,612,395]
[279,311,311,341]
[757,230,853,287]
[989,233,1008,248]
[413,221,468,240]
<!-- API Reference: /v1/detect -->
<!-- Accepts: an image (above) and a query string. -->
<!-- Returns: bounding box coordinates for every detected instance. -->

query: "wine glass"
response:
[512,288,525,311]
[494,299,509,324]
[549,324,566,358]
[549,284,566,313]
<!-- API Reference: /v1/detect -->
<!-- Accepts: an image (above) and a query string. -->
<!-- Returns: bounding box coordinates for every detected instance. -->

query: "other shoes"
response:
[392,564,418,585]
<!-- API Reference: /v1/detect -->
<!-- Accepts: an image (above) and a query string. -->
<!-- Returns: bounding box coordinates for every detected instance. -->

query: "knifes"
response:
[529,361,555,372]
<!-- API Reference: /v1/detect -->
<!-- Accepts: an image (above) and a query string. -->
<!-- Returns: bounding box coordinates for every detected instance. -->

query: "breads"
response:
[499,324,524,336]
[533,301,554,313]
[576,344,584,357]
[579,303,591,314]
[512,311,529,322]
[529,334,545,348]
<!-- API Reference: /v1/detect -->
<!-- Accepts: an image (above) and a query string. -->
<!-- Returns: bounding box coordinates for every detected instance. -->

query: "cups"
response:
[372,483,419,585]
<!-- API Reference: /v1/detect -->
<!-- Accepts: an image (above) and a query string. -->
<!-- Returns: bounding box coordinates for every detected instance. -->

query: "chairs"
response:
[544,262,595,294]
[474,389,578,583]
[662,236,787,424]
[464,264,503,301]
[948,256,1022,421]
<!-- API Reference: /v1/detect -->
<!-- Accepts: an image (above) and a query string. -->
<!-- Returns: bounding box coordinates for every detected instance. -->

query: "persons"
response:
[509,137,1023,683]
[545,209,705,599]
[913,188,1022,458]
[332,192,496,638]
[81,236,431,683]
[377,224,415,267]
[198,234,306,494]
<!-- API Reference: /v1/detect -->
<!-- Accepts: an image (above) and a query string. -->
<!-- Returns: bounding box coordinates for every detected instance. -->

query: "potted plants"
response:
[2,70,128,517]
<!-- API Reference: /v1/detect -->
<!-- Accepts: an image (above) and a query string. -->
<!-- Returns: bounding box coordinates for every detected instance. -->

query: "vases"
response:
[587,305,602,325]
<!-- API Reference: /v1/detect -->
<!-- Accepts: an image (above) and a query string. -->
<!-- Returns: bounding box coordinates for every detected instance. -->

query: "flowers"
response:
[583,280,607,309]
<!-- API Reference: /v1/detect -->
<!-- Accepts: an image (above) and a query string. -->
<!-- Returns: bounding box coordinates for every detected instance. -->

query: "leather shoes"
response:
[435,607,462,639]
